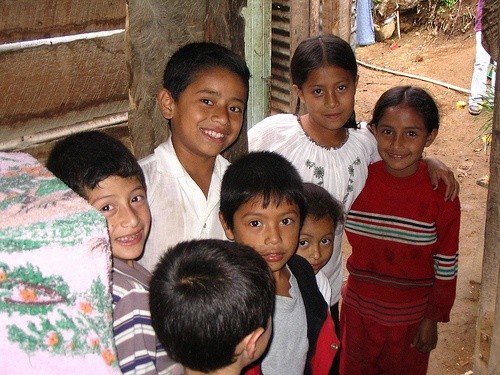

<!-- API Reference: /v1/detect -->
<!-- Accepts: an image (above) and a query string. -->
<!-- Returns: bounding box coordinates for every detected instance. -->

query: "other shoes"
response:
[468,102,482,116]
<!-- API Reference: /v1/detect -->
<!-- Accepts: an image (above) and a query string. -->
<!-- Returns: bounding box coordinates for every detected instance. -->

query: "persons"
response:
[48,129,185,375]
[341,85,461,375]
[295,182,345,305]
[134,42,252,272]
[219,151,340,375]
[249,35,460,375]
[468,0,496,114]
[148,238,275,375]
